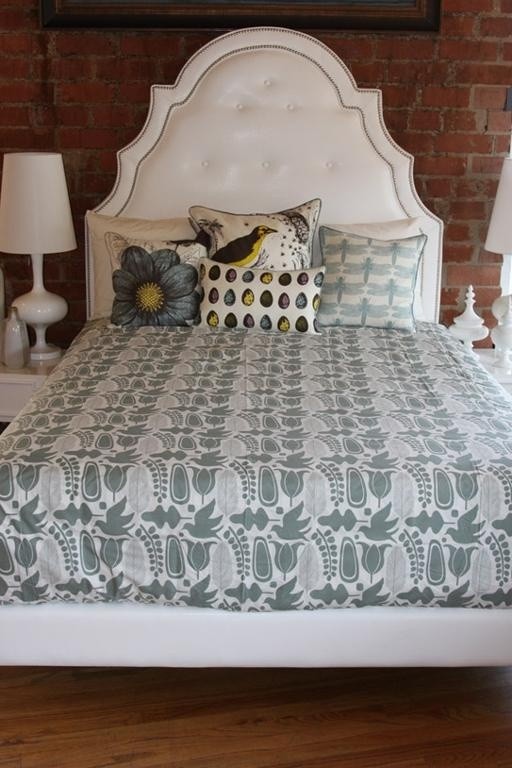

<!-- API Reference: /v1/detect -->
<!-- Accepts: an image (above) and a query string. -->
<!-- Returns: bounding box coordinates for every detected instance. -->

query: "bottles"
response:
[0,306,30,370]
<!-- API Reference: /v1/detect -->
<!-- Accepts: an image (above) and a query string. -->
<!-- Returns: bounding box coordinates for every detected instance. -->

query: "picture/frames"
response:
[39,0,440,33]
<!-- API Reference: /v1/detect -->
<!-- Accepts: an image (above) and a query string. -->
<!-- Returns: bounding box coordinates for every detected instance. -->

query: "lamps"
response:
[483,154,510,370]
[0,152,77,361]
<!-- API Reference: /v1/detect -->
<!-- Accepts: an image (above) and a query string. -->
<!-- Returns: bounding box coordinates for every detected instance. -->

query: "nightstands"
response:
[476,349,510,397]
[0,350,66,423]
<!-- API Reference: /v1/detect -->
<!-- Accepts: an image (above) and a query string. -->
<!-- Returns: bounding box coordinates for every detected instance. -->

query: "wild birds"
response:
[212,224,277,267]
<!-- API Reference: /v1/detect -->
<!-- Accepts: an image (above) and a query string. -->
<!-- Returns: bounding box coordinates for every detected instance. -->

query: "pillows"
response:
[103,229,211,332]
[187,197,323,269]
[320,213,426,325]
[319,228,428,335]
[198,255,326,335]
[86,208,203,317]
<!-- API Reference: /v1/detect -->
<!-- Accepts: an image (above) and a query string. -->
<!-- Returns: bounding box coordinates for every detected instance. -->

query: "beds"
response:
[0,27,511,668]
